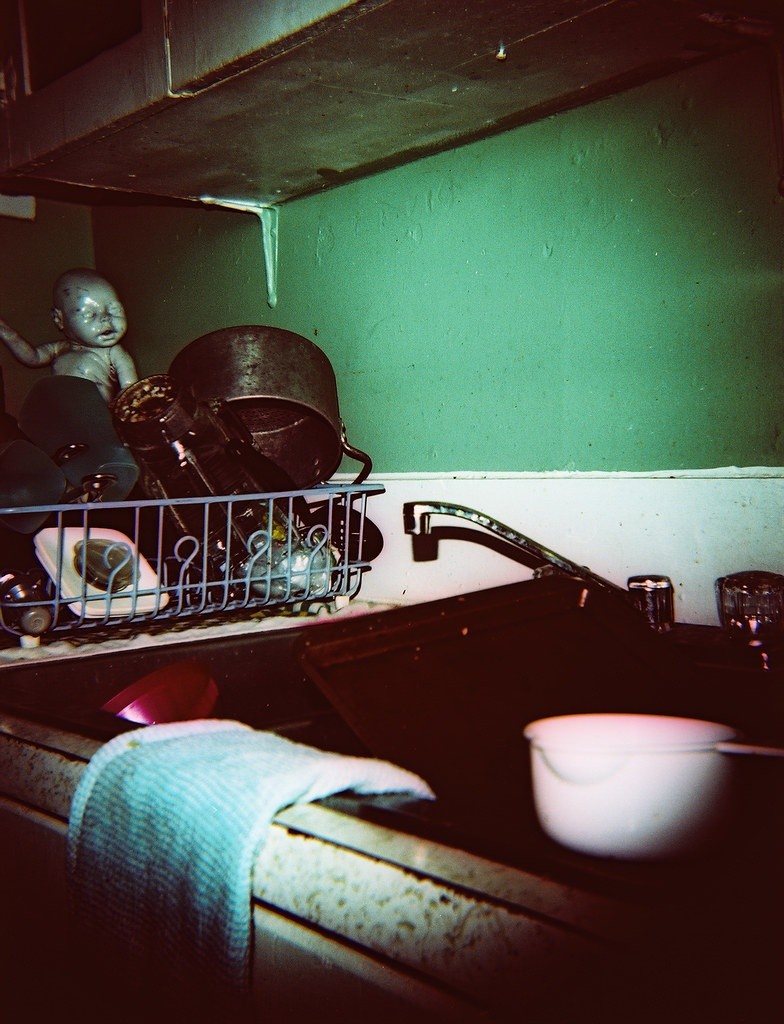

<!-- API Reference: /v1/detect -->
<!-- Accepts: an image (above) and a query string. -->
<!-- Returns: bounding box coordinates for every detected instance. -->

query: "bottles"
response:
[0,567,51,635]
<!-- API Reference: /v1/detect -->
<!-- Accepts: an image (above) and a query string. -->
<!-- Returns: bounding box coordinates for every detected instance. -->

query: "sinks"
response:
[0,576,784,974]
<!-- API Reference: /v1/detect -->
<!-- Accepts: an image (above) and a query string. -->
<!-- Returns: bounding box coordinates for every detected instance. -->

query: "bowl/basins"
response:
[102,660,218,726]
[523,714,784,858]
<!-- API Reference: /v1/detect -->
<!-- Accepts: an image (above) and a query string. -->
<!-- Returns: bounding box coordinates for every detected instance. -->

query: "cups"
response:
[0,375,140,535]
[110,373,299,582]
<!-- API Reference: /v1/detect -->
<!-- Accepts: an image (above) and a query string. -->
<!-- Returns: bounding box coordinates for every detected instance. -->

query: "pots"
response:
[171,326,372,488]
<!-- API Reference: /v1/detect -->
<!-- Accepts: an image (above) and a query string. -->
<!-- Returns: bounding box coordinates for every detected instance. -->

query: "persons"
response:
[0,268,138,404]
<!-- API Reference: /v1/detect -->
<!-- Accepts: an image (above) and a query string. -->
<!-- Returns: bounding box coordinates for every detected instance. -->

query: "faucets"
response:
[402,500,678,638]
